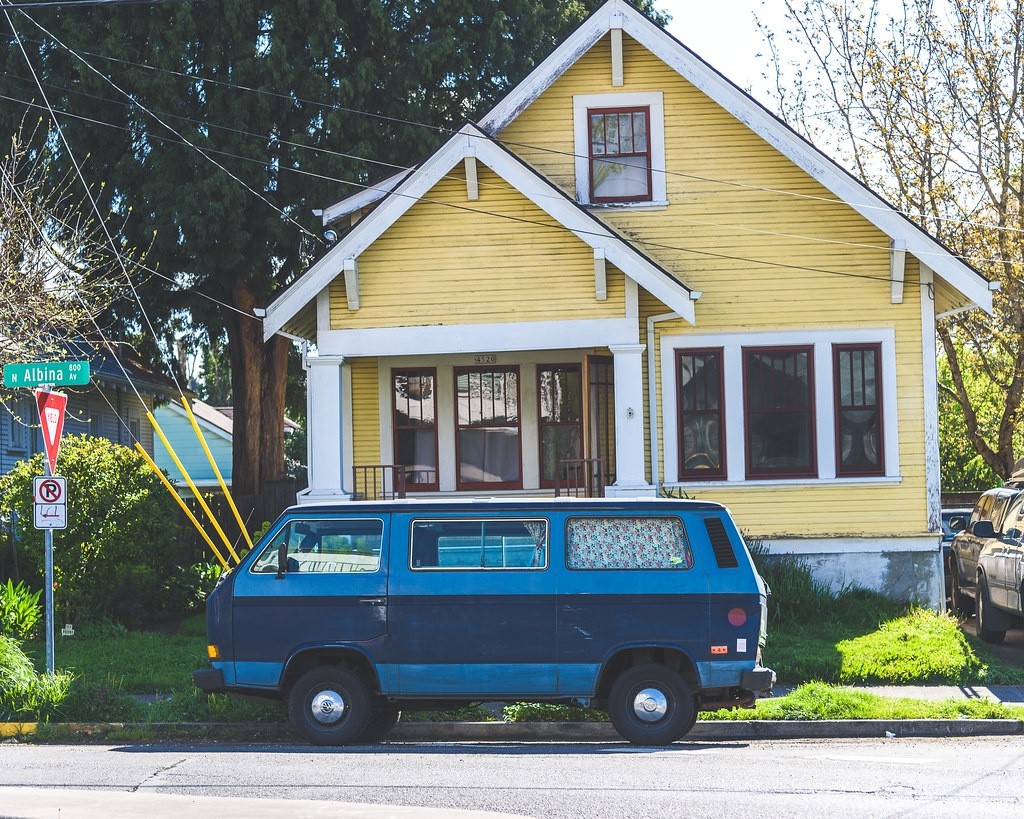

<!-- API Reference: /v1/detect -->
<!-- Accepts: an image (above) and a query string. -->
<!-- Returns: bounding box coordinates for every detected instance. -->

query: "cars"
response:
[940,466,1024,646]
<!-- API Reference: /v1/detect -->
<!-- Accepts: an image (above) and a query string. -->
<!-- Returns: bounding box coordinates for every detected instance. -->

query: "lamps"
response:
[323,230,338,246]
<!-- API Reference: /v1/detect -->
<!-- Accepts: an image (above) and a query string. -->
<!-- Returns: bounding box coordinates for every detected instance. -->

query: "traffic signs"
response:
[33,476,67,530]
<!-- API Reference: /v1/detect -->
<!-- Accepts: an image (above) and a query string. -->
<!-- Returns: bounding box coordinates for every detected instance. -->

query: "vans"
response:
[191,497,777,745]
